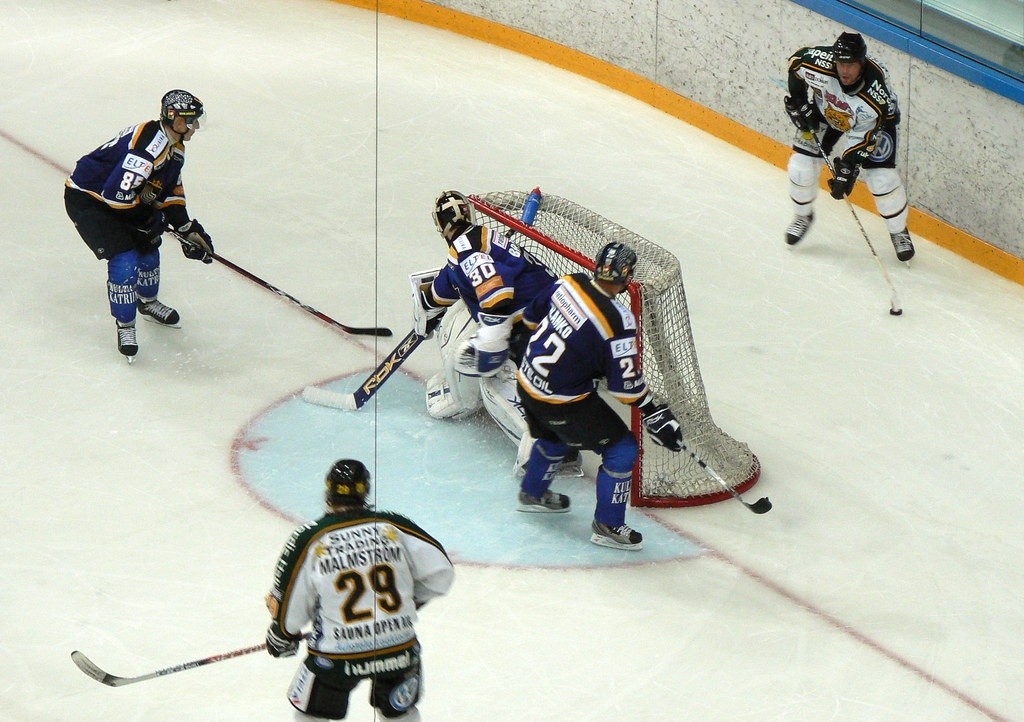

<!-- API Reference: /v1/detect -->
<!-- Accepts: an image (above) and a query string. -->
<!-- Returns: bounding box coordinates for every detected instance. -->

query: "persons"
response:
[515,241,683,552]
[64,89,214,365]
[265,459,455,722]
[785,32,916,267]
[409,191,584,479]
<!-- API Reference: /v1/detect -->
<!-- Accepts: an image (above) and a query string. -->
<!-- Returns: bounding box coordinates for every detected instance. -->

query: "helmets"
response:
[326,460,371,507]
[160,89,204,122]
[431,190,474,235]
[595,241,638,283]
[832,32,867,62]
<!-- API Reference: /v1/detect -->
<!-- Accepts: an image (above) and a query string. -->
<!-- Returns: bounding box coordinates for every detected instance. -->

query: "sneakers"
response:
[137,296,182,329]
[517,486,569,513]
[783,213,815,248]
[590,517,643,551]
[115,318,139,364]
[891,227,915,269]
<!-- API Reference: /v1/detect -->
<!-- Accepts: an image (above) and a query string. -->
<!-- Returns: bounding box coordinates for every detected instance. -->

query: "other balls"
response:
[890,309,902,316]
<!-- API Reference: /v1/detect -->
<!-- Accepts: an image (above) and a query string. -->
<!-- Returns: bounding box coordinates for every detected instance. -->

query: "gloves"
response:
[175,219,214,263]
[137,207,169,235]
[828,162,861,200]
[784,95,821,131]
[642,404,682,451]
[266,620,303,657]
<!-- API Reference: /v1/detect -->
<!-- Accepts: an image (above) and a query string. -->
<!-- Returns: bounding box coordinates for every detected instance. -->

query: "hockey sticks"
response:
[164,227,392,337]
[71,631,312,687]
[301,316,443,411]
[676,439,773,514]
[808,124,899,312]
